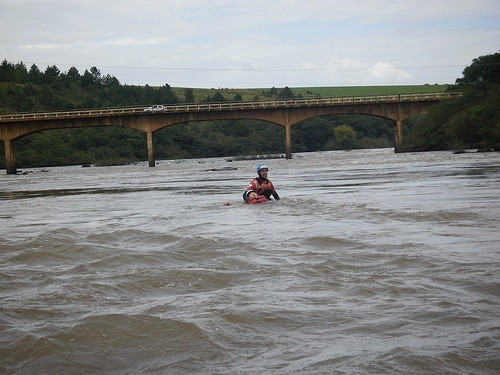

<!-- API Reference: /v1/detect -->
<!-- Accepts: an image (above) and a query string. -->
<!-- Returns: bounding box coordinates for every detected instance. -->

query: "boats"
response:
[453,142,478,154]
[82,164,90,167]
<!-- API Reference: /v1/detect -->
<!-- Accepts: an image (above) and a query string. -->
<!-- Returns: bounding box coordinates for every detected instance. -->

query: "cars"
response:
[143,105,167,112]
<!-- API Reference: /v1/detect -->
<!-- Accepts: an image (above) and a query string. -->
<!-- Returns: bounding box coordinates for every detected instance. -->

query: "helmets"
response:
[243,189,254,200]
[257,165,269,173]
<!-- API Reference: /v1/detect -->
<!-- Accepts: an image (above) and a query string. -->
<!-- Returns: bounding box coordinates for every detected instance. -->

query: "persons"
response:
[242,163,281,204]
